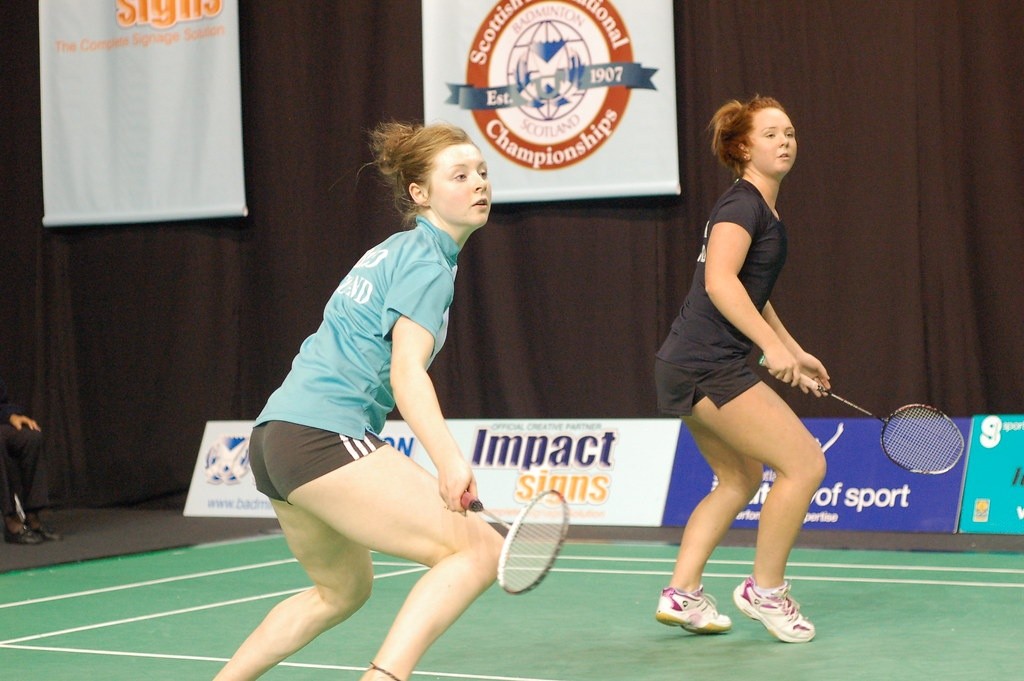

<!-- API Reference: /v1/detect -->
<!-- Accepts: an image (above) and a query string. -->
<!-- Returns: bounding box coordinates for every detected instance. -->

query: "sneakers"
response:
[656,584,732,634]
[733,576,815,642]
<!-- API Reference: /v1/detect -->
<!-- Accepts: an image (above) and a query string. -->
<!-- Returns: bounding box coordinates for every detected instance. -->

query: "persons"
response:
[648,92,831,646]
[206,121,512,681]
[0,401,65,546]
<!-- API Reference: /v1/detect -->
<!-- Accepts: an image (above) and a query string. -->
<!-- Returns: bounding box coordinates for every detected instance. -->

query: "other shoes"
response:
[4,522,65,545]
